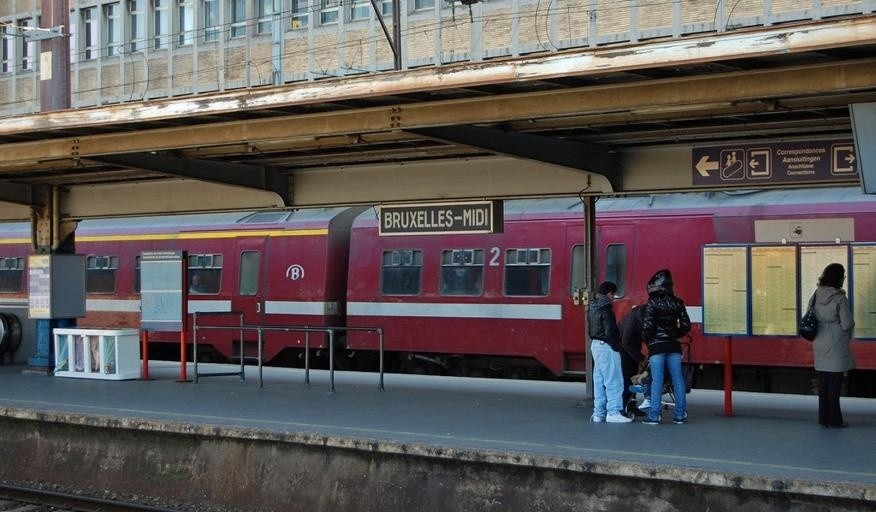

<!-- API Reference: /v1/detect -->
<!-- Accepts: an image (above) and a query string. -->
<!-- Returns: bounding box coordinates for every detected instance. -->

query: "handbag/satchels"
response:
[798,289,819,342]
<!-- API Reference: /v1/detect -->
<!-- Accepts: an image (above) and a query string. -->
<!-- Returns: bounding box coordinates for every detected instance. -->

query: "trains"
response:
[0,201,876,382]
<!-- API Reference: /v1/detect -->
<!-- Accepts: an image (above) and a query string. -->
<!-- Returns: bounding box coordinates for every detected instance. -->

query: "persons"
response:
[586,281,632,424]
[640,267,692,426]
[616,302,650,418]
[629,352,652,410]
[805,262,857,429]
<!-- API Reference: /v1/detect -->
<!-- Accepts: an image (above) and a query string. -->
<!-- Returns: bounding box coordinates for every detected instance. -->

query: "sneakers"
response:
[821,421,849,429]
[593,384,686,425]
